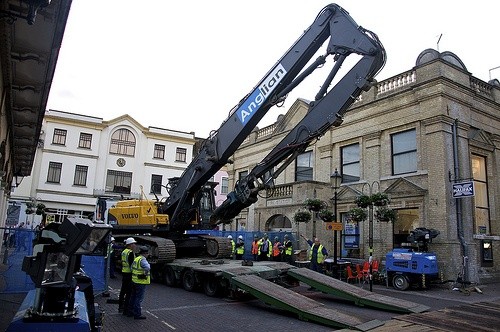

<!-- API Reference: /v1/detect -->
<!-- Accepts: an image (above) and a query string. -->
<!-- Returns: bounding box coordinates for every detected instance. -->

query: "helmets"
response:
[139,246,148,253]
[275,236,279,242]
[126,237,137,244]
[254,235,258,238]
[238,235,243,239]
[110,237,115,241]
[227,235,232,239]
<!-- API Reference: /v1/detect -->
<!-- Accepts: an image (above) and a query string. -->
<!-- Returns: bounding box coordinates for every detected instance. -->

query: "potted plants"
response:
[371,192,390,207]
[346,208,368,223]
[354,195,370,208]
[374,208,398,224]
[295,212,312,225]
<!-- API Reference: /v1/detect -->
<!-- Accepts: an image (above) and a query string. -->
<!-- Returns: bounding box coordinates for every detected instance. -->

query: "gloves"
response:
[141,274,146,279]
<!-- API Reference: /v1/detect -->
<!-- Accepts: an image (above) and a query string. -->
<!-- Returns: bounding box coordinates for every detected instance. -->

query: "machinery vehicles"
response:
[385,227,443,292]
[103,2,389,260]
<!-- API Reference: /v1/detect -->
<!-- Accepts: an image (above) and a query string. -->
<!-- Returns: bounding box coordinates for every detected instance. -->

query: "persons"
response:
[227,234,244,259]
[104,236,151,320]
[300,234,329,274]
[250,233,292,264]
[18,220,45,254]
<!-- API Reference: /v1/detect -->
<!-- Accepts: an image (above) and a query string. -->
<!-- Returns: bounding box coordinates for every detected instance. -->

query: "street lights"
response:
[25,196,45,255]
[330,166,342,284]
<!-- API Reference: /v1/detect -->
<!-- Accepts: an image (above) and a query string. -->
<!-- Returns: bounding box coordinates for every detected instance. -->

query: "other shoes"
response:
[128,313,134,317]
[123,311,128,315]
[119,310,123,313]
[308,287,319,291]
[134,315,146,319]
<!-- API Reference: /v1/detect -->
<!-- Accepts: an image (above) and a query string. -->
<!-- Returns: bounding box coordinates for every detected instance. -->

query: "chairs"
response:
[348,259,378,285]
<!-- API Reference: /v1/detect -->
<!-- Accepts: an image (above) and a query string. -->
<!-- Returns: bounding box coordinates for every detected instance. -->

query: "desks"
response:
[295,256,350,280]
